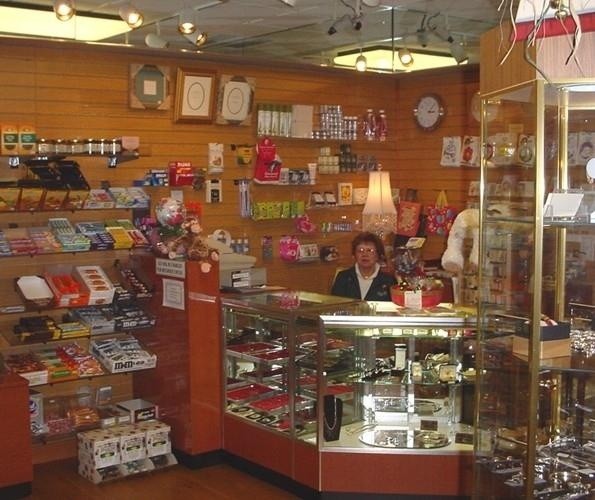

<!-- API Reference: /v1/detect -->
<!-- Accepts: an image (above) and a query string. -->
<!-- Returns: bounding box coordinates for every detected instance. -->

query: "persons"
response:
[330,232,399,302]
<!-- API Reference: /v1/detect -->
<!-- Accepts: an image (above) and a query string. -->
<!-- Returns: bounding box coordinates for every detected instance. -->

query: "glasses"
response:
[359,248,376,254]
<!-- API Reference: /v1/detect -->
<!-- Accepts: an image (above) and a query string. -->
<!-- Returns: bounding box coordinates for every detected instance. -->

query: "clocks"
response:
[414,92,445,132]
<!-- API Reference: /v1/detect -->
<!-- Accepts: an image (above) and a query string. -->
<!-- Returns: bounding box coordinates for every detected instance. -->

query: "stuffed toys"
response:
[170,216,223,274]
[149,196,189,259]
[440,209,494,273]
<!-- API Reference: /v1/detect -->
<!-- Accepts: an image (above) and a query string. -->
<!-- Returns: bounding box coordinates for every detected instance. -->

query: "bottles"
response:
[364,109,387,143]
[230,239,249,253]
[312,104,358,140]
[257,103,291,138]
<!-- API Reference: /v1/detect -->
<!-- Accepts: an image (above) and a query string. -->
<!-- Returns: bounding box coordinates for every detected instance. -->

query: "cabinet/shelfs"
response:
[478,19,595,500]
[1,148,156,466]
[0,366,34,500]
[251,131,398,268]
[220,284,558,500]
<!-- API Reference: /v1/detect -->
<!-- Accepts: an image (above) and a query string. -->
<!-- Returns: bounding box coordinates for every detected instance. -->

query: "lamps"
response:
[182,28,206,47]
[352,21,361,30]
[356,46,366,70]
[145,22,170,50]
[53,1,76,21]
[363,164,398,263]
[417,28,428,48]
[179,6,196,33]
[1,1,133,43]
[448,37,469,67]
[118,3,144,29]
[333,45,468,76]
[329,14,352,34]
[398,38,413,68]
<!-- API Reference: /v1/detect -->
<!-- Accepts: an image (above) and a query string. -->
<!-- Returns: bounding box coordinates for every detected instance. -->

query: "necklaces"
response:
[324,396,338,430]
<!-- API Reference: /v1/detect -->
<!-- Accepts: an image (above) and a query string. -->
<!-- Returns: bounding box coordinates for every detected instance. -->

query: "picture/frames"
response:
[173,65,220,125]
[214,72,256,127]
[127,62,173,111]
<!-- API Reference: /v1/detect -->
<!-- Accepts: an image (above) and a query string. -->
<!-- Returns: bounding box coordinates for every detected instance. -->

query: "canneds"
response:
[36,138,122,155]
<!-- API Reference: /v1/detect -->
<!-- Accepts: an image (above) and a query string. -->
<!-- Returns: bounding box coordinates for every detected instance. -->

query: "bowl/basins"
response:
[390,283,443,306]
